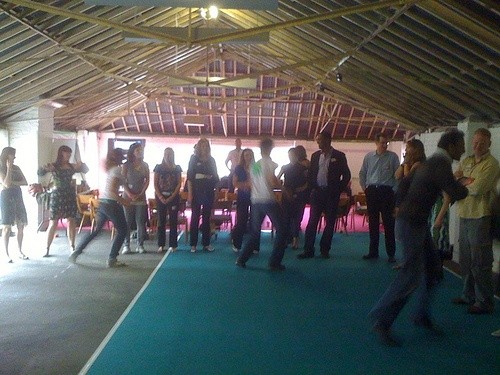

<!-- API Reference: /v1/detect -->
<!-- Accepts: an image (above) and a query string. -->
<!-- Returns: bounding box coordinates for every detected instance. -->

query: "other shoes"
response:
[369,319,402,347]
[204,246,215,251]
[69,251,77,263]
[43,254,48,257]
[19,255,28,260]
[388,257,396,264]
[122,246,131,254]
[296,252,313,258]
[158,246,164,252]
[362,253,379,260]
[106,258,125,267]
[236,260,246,267]
[136,246,146,253]
[321,251,329,257]
[408,310,440,335]
[468,306,485,314]
[170,248,176,253]
[191,246,196,253]
[269,264,285,270]
[8,259,12,263]
[452,297,465,305]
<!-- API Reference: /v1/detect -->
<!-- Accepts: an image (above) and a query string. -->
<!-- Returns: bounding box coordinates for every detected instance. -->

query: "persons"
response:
[153,147,183,254]
[224,131,351,271]
[187,135,219,253]
[121,143,150,255]
[37,144,90,257]
[0,147,28,263]
[358,128,500,347]
[68,147,129,267]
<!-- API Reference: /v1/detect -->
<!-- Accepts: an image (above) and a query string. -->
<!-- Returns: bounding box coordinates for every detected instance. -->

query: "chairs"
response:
[271,189,383,241]
[76,189,236,244]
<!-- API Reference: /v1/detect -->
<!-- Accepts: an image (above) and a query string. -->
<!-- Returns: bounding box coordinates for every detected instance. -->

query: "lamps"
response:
[336,73,342,81]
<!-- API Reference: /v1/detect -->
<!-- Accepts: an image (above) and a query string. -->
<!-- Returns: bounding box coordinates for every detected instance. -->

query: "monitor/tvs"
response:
[108,138,145,164]
